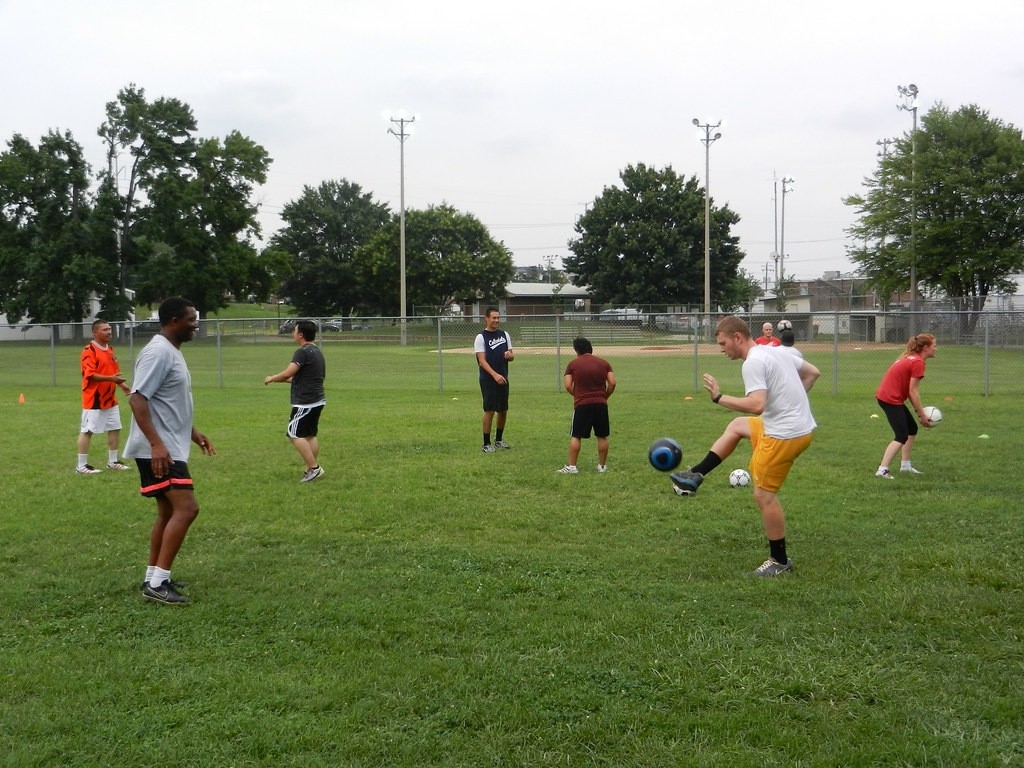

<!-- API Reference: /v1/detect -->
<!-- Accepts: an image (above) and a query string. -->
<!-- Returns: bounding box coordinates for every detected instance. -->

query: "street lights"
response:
[780,177,794,282]
[895,85,919,343]
[387,114,417,342]
[692,118,722,341]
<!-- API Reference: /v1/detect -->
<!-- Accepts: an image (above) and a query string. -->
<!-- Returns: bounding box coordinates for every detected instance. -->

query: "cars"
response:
[279,319,361,335]
[125,319,160,338]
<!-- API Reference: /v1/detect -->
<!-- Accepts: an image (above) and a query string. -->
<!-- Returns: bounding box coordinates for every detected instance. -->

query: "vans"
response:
[600,309,642,321]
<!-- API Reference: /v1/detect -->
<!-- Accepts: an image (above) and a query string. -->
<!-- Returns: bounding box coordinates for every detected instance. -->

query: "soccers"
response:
[777,319,792,333]
[649,437,684,473]
[918,406,941,428]
[729,468,750,487]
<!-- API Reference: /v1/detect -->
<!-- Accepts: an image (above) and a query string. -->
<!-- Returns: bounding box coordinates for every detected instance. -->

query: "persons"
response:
[473,307,515,453]
[265,320,327,483]
[74,319,132,474]
[556,337,617,474]
[122,296,216,606]
[875,334,937,479]
[669,316,821,577]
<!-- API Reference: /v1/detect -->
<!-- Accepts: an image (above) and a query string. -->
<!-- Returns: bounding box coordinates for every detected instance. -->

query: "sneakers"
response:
[899,466,923,474]
[107,460,133,470]
[494,438,510,450]
[300,465,324,483]
[482,444,496,452]
[557,465,578,474]
[875,470,895,480]
[597,464,607,473]
[753,553,793,577]
[669,468,704,491]
[75,464,103,474]
[141,577,192,605]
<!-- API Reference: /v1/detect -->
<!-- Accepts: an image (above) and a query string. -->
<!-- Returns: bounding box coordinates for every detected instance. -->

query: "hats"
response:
[781,330,794,346]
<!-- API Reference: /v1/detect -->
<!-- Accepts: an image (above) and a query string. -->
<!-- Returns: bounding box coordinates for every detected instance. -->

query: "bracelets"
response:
[713,393,723,404]
